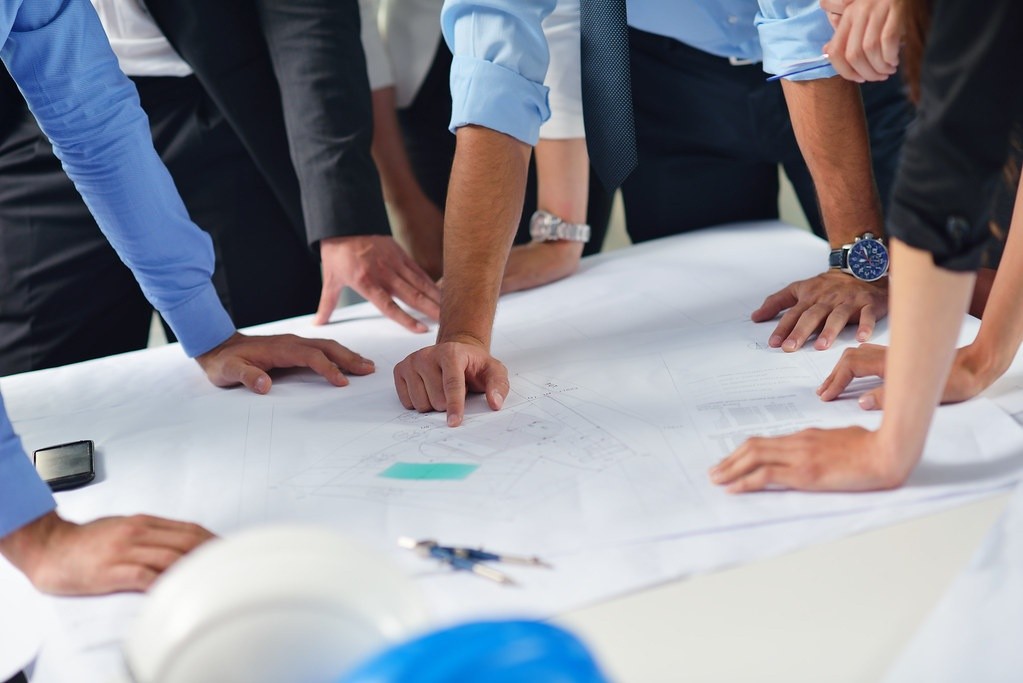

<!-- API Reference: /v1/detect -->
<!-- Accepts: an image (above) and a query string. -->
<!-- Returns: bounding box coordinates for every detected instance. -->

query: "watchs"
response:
[529,209,592,244]
[829,232,890,282]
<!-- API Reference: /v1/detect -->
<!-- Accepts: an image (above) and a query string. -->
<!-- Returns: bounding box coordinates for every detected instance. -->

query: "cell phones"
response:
[34,440,94,492]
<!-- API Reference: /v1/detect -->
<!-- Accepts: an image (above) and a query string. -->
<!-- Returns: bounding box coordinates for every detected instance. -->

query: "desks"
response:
[0,220,1022,683]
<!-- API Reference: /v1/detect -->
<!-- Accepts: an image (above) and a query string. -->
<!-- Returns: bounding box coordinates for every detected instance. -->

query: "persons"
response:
[817,0,1021,321]
[394,0,920,427]
[1,0,376,598]
[705,0,1021,491]
[0,0,440,375]
[348,0,616,301]
